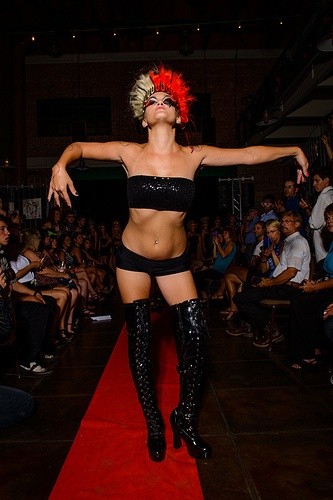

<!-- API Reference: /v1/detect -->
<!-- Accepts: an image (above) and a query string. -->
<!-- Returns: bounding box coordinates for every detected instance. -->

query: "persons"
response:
[0,135,333,429]
[48,65,309,462]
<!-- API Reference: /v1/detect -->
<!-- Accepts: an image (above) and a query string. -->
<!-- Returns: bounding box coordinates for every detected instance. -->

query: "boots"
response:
[170,296,213,459]
[120,298,167,462]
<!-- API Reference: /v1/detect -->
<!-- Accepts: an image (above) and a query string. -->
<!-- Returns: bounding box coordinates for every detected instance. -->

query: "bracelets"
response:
[260,259,267,263]
[33,289,39,297]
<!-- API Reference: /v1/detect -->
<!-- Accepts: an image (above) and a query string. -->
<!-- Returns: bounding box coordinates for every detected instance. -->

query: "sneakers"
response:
[226,325,253,338]
[253,328,283,347]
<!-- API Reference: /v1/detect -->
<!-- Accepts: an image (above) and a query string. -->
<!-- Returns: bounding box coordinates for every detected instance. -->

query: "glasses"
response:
[279,220,300,226]
[264,229,279,236]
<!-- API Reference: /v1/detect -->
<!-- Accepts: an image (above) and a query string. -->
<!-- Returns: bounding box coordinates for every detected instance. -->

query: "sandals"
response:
[289,357,320,371]
[219,307,231,315]
[222,308,239,321]
[209,293,224,301]
[79,308,96,317]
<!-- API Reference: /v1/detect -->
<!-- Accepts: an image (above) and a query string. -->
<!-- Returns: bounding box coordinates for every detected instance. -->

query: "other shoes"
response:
[99,285,114,295]
[86,305,96,310]
[56,332,72,342]
[65,324,75,335]
[45,352,60,358]
[91,293,106,305]
[37,364,55,374]
[200,296,208,304]
[20,361,46,373]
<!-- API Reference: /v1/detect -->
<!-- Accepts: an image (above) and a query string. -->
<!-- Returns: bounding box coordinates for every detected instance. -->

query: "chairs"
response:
[259,300,290,352]
